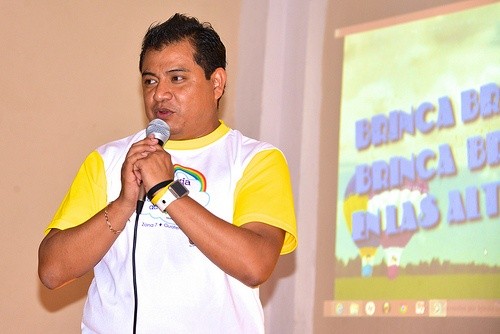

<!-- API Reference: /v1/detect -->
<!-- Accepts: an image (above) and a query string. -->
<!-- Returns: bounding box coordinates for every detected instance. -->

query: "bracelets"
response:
[147,180,176,206]
[104,201,130,234]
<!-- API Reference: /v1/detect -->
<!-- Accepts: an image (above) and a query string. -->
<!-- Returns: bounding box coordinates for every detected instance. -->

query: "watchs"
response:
[156,179,189,213]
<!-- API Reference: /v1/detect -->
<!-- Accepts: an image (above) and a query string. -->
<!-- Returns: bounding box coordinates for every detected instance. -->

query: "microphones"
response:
[136,119,170,214]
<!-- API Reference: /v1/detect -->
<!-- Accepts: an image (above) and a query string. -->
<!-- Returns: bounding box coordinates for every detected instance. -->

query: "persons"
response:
[38,13,300,334]
